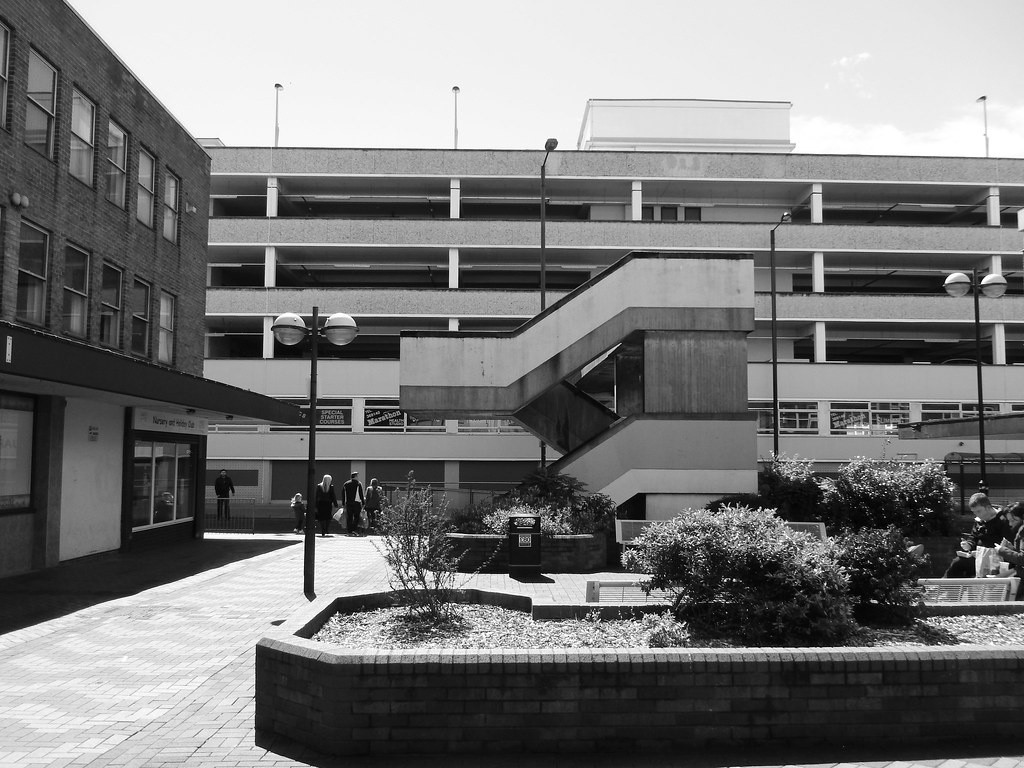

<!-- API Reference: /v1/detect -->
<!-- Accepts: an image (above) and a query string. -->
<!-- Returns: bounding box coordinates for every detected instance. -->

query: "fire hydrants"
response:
[269,303,360,605]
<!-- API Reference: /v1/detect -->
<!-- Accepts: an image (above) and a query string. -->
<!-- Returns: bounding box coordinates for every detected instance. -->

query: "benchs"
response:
[617,520,830,553]
[917,577,1021,602]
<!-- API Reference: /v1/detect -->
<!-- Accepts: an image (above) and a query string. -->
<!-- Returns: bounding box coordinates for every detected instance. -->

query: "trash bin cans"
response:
[508,514,542,578]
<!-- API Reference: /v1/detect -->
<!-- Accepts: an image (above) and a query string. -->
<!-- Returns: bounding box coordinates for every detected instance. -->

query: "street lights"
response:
[273,84,283,146]
[976,93,989,154]
[536,138,558,469]
[451,86,460,148]
[769,210,792,466]
[940,272,1008,499]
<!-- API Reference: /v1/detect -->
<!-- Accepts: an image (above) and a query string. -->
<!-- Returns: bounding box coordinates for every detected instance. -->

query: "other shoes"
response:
[294,528,305,534]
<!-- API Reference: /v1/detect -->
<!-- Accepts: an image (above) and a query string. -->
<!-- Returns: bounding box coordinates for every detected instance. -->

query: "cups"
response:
[1000,562,1009,574]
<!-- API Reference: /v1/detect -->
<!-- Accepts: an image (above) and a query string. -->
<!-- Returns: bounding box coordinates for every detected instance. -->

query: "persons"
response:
[290,493,306,534]
[364,478,384,534]
[942,493,1024,602]
[314,474,339,538]
[341,472,366,537]
[214,469,235,520]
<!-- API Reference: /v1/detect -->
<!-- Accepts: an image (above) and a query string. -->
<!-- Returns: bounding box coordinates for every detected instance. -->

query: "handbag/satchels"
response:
[314,511,318,522]
[355,481,362,503]
[357,507,369,530]
[333,507,347,529]
